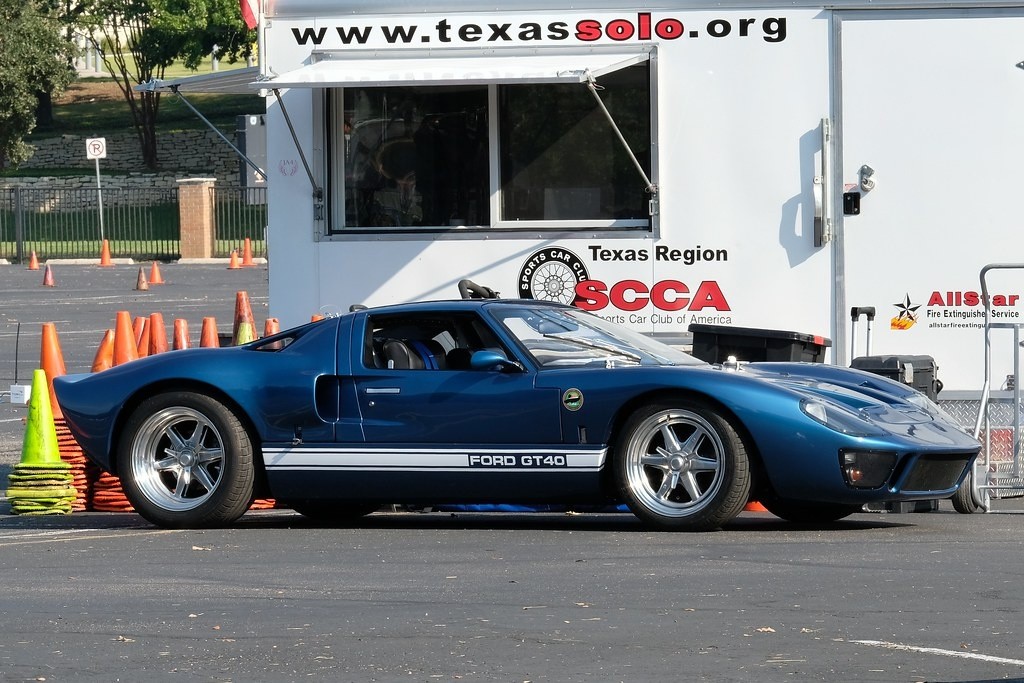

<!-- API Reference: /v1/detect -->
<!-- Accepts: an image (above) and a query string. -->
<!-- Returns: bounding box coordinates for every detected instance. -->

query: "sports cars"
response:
[53,300,981,531]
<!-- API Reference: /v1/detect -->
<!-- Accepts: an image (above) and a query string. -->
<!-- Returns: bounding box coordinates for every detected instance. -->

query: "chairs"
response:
[450,347,507,370]
[383,339,447,370]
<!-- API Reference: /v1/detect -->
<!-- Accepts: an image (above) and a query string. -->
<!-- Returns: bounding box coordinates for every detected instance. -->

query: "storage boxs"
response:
[688,324,832,364]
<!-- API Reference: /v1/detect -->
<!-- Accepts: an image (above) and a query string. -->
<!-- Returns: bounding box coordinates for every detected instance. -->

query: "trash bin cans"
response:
[687,322,834,514]
[850,353,939,516]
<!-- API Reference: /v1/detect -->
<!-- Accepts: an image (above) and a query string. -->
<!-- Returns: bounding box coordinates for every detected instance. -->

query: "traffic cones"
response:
[30,239,255,290]
[4,292,323,515]
